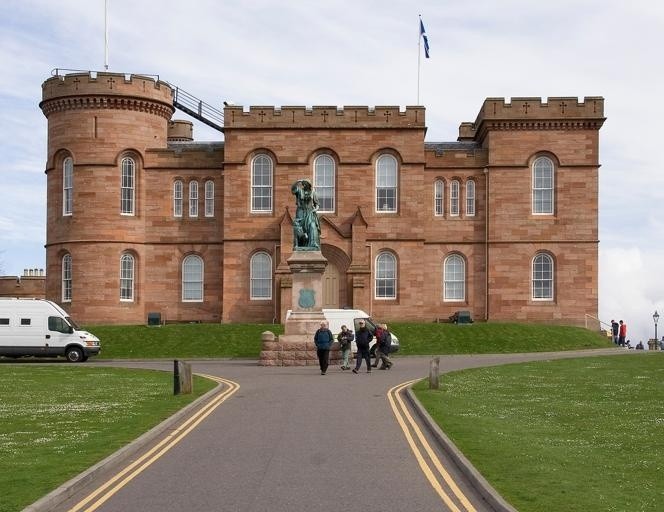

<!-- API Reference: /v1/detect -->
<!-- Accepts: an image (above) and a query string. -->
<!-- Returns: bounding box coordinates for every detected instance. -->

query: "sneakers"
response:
[321,371,327,375]
[371,364,377,367]
[352,368,360,374]
[378,362,394,370]
[367,368,372,373]
[341,366,351,370]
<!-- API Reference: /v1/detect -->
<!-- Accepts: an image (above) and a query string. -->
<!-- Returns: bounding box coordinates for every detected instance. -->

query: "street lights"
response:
[653,310,659,350]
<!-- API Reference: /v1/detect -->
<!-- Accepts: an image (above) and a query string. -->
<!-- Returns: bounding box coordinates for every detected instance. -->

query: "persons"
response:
[369,323,393,371]
[659,335,664,350]
[288,178,320,250]
[313,320,334,375]
[617,320,626,346]
[624,339,644,350]
[351,320,373,374]
[336,324,354,371]
[610,319,619,344]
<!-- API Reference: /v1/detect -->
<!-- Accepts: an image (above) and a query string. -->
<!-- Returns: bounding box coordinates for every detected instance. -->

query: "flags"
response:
[419,18,430,59]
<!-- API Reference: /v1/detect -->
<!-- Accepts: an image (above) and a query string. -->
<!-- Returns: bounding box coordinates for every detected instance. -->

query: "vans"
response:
[0,297,101,362]
[285,307,400,357]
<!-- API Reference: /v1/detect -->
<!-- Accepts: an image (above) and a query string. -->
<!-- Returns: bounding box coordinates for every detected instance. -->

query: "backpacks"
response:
[380,331,391,346]
